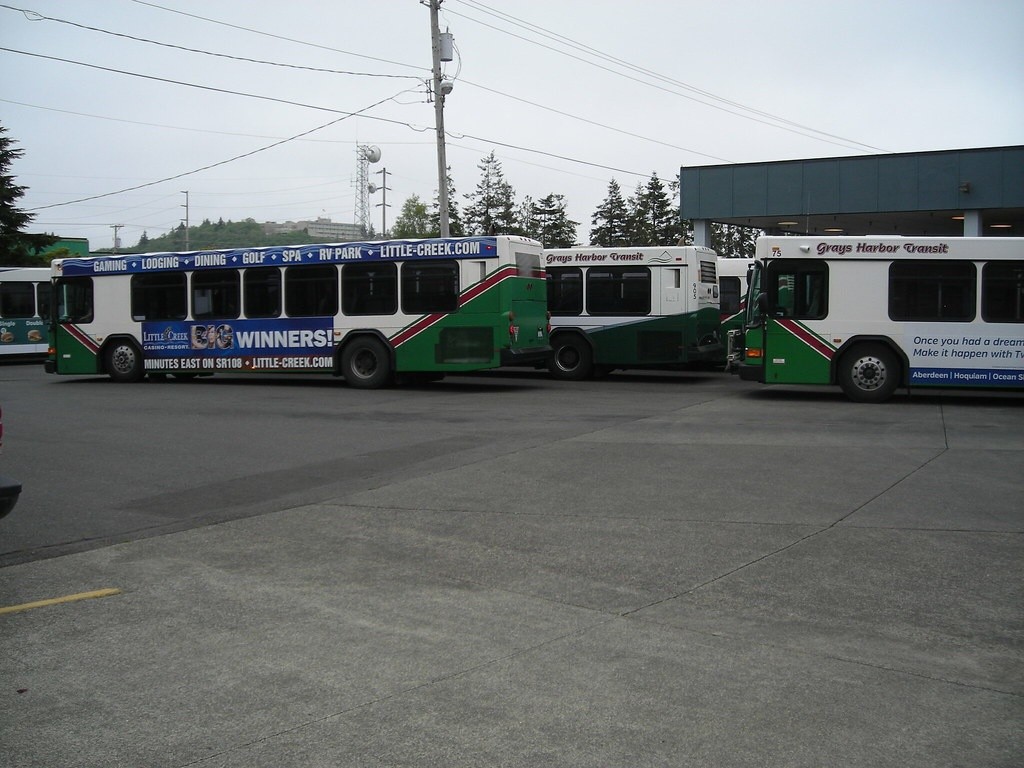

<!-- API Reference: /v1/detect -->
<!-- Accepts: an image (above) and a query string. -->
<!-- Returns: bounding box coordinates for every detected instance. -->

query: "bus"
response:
[716,255,797,371]
[542,246,722,381]
[0,267,52,363]
[44,235,553,388]
[726,234,1024,401]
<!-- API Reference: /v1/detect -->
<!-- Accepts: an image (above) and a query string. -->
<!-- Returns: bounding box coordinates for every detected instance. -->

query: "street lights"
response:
[434,81,453,238]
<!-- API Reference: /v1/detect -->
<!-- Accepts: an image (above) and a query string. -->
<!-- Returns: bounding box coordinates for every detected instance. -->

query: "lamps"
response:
[958,181,971,194]
